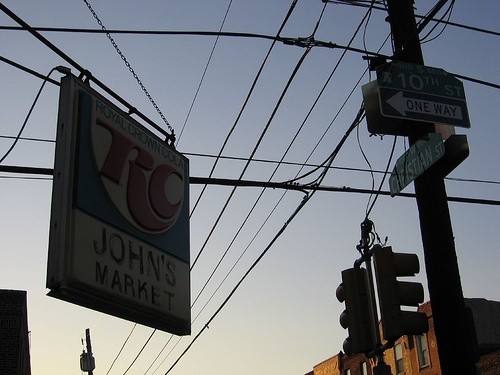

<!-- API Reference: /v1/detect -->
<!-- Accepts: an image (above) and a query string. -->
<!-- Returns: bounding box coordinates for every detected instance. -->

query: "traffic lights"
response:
[373,244,429,338]
[336,267,379,356]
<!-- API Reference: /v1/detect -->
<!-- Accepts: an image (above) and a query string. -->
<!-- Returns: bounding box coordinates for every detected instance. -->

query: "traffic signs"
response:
[389,133,445,197]
[376,63,470,129]
